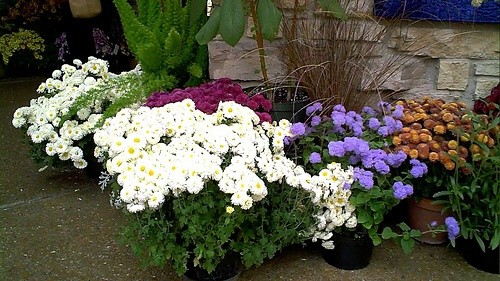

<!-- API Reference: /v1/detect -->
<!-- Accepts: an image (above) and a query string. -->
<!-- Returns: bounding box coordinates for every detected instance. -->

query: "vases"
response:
[178,233,243,281]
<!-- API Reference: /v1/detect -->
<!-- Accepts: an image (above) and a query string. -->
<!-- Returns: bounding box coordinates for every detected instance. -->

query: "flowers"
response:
[0,0,500,276]
